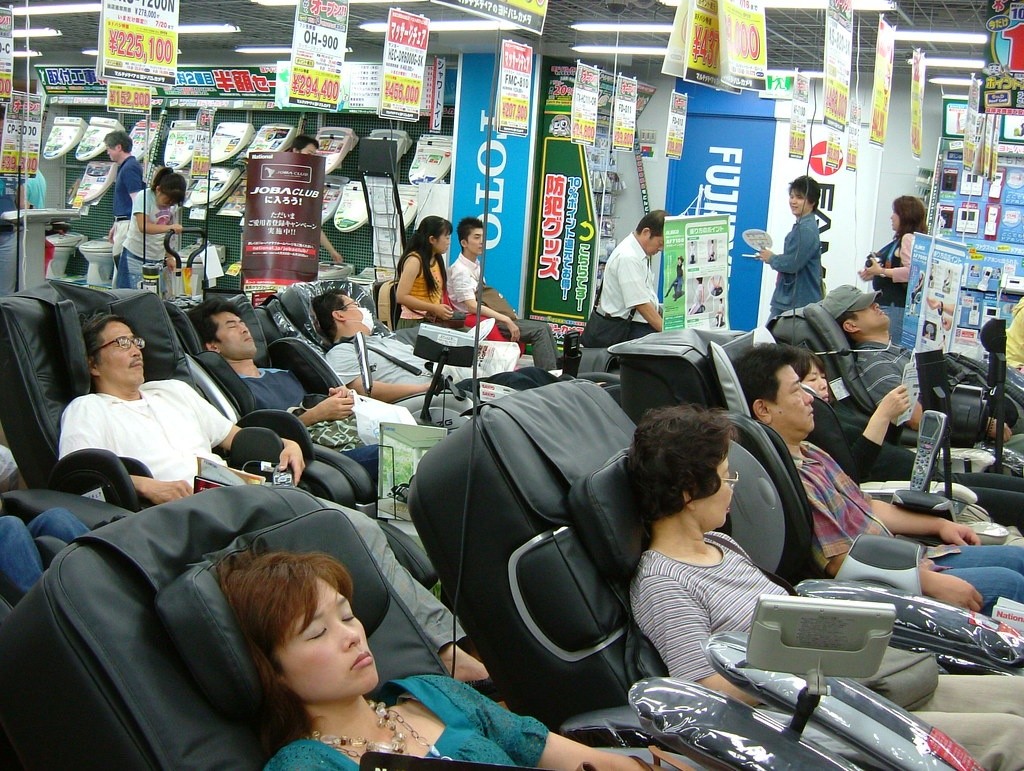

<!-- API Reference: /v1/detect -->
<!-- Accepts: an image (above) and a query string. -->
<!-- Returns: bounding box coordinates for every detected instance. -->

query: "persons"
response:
[219,552,676,771]
[25,164,47,209]
[0,443,91,596]
[104,130,148,289]
[114,167,187,288]
[290,135,344,265]
[731,342,1024,611]
[1006,295,1024,375]
[627,401,1024,771]
[187,296,380,480]
[857,196,929,307]
[57,309,508,703]
[594,209,670,342]
[820,284,1024,458]
[312,216,562,406]
[754,176,825,328]
[780,340,1024,536]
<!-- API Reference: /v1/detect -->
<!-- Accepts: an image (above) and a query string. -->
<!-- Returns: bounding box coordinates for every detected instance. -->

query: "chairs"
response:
[0,280,1024,771]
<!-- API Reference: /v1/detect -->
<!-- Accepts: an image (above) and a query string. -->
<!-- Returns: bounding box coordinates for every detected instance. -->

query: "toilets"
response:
[318,261,355,279]
[78,236,113,285]
[171,240,226,296]
[45,232,88,279]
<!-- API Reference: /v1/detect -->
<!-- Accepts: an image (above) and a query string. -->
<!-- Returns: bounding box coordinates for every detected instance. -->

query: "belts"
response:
[113,216,129,222]
[0,224,14,231]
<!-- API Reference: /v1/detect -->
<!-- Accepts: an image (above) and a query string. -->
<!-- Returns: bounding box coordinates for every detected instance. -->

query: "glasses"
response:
[89,336,146,356]
[720,471,739,490]
[338,299,359,312]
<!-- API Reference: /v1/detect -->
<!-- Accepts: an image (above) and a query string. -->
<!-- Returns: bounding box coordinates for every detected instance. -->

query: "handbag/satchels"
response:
[426,307,465,329]
[580,308,632,348]
[351,389,418,446]
[474,281,516,322]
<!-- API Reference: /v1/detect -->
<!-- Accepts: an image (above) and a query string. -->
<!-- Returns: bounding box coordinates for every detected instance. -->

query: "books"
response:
[377,421,449,523]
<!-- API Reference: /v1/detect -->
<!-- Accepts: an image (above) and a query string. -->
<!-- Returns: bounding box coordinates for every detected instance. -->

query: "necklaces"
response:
[300,699,452,762]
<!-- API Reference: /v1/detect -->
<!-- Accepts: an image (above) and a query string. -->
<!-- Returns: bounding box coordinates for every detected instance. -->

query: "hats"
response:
[822,284,883,319]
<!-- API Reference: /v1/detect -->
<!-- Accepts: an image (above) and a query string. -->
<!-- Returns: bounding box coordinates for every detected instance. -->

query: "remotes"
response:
[354,332,373,395]
[910,410,948,492]
[273,465,292,487]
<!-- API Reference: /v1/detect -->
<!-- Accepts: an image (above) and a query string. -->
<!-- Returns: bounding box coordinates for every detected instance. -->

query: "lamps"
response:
[766,67,824,79]
[177,22,242,34]
[905,54,987,69]
[8,2,182,58]
[927,75,984,87]
[232,43,354,54]
[894,28,988,45]
[568,42,667,55]
[357,17,522,33]
[563,19,673,33]
